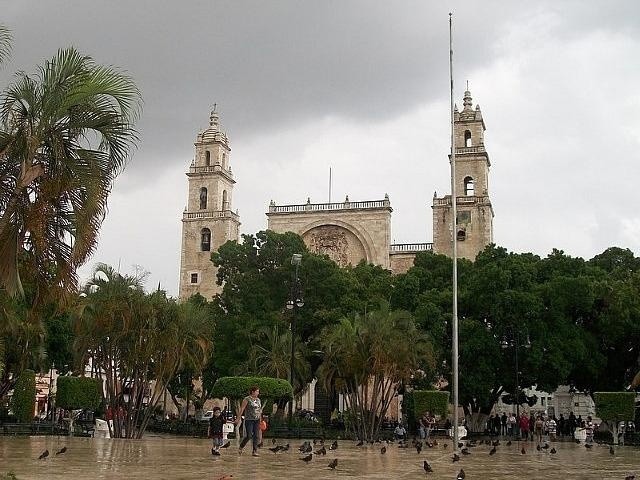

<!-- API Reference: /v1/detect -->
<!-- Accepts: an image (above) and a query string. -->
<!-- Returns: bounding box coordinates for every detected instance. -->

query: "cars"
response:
[33,411,51,424]
[618,421,635,433]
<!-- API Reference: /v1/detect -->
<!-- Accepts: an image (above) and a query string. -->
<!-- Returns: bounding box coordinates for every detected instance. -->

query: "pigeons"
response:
[55,446,67,455]
[211,447,219,456]
[455,469,467,480]
[326,458,338,471]
[264,433,341,464]
[220,441,231,452]
[355,435,558,462]
[609,446,615,455]
[584,443,593,450]
[422,460,435,474]
[574,438,580,444]
[625,475,636,480]
[37,449,50,460]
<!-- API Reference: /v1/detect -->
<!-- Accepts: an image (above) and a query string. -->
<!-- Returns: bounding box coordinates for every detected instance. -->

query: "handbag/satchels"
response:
[260,420,267,431]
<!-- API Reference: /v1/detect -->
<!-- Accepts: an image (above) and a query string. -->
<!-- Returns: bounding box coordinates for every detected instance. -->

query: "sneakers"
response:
[211,447,220,457]
[237,449,259,456]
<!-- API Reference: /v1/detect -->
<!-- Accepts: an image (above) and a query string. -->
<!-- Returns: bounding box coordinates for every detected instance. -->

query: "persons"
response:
[585,416,596,442]
[105,405,114,421]
[419,411,435,439]
[236,387,262,456]
[444,419,451,437]
[394,424,407,440]
[208,406,236,455]
[170,413,176,419]
[116,406,128,432]
[488,411,586,442]
[222,407,227,420]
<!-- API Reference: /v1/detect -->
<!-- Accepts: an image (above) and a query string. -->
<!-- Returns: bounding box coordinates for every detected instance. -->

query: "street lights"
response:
[499,332,532,441]
[286,253,304,438]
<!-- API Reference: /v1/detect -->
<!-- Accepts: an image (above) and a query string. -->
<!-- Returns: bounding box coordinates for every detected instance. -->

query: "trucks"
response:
[201,410,233,423]
